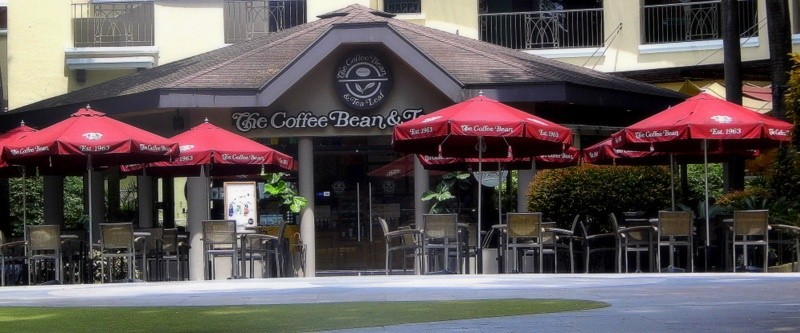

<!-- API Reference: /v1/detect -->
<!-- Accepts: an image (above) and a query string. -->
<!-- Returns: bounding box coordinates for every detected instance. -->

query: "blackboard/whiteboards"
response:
[224,181,258,234]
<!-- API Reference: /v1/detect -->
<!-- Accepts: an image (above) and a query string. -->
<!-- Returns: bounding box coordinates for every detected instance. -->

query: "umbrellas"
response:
[3,102,180,285]
[582,136,761,273]
[612,92,795,273]
[415,146,580,274]
[389,88,574,276]
[118,117,294,283]
[0,120,39,287]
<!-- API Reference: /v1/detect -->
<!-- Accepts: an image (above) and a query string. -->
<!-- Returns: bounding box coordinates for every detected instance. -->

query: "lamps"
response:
[76,70,85,84]
[172,109,185,131]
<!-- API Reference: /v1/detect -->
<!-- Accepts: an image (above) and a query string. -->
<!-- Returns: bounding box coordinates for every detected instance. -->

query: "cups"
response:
[315,219,329,228]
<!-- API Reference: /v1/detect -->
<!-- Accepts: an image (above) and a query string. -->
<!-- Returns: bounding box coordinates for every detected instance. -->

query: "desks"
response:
[213,230,255,279]
[411,222,468,274]
[34,235,80,284]
[724,217,763,272]
[109,231,153,282]
[649,218,662,272]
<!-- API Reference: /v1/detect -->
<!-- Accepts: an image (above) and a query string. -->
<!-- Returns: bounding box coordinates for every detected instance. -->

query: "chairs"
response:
[1,208,799,285]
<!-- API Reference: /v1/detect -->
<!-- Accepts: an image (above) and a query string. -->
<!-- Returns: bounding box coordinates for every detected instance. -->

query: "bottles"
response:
[372,216,397,228]
